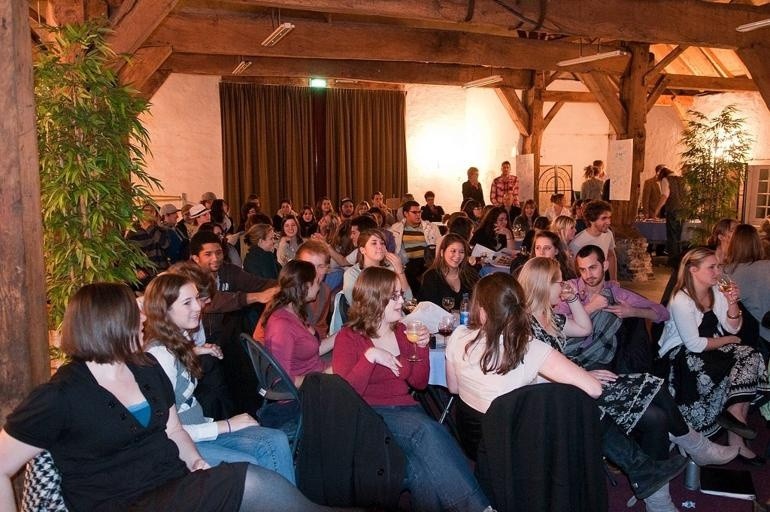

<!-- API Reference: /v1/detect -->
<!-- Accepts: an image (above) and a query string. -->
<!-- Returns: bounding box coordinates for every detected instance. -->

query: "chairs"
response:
[339,295,350,323]
[239,331,302,466]
[292,372,406,510]
[477,382,608,511]
[424,244,436,258]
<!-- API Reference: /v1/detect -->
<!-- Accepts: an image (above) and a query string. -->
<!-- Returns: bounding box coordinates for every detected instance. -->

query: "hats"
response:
[159,204,182,215]
[189,204,212,219]
[199,192,218,202]
[339,197,354,204]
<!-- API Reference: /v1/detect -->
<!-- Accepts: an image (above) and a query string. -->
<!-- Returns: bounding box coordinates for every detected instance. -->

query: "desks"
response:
[479,261,513,277]
[412,313,466,445]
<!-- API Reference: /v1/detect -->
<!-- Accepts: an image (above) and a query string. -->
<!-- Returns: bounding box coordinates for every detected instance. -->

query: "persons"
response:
[0,158,769,512]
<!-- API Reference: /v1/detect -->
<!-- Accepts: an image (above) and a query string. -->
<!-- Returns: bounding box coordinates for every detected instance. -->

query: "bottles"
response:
[460,293,471,325]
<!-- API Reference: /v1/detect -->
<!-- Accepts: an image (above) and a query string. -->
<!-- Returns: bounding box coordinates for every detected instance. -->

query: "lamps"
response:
[557,51,626,69]
[736,18,770,33]
[261,23,294,46]
[232,62,252,75]
[462,74,503,89]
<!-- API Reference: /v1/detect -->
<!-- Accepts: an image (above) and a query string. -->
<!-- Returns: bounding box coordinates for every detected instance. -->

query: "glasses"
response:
[555,279,564,286]
[408,209,424,214]
[215,232,226,238]
[383,292,406,300]
[474,207,483,211]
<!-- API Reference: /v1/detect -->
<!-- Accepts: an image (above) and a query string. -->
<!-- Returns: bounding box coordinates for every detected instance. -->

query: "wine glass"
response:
[438,317,454,348]
[442,297,455,313]
[561,283,587,299]
[717,276,742,304]
[402,298,417,313]
[406,321,422,362]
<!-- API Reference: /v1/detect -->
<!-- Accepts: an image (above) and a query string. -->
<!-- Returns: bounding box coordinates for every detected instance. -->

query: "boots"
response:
[585,411,690,501]
[668,425,741,468]
[644,480,679,512]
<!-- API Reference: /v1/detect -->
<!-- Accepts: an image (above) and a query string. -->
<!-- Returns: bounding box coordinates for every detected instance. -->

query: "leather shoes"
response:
[716,410,758,439]
[725,443,762,467]
